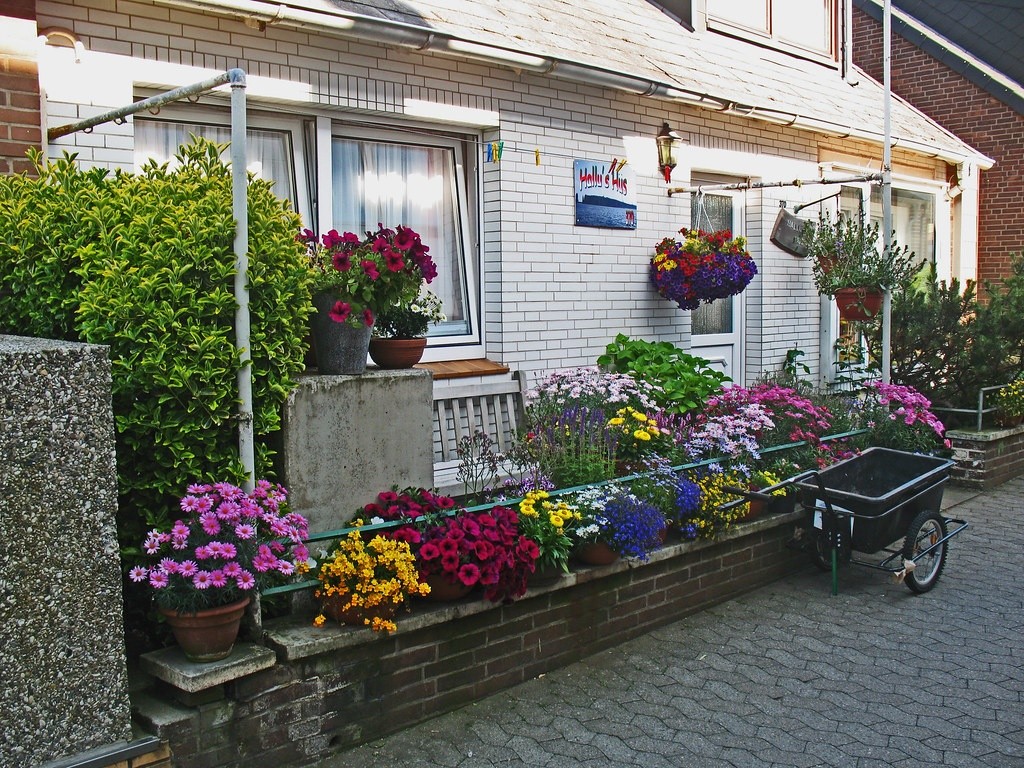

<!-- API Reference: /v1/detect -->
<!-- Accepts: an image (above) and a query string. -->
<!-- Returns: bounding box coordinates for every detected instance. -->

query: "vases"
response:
[648,524,667,546]
[160,596,252,662]
[574,535,619,566]
[323,594,404,626]
[727,483,764,522]
[767,480,795,512]
[991,412,1024,428]
[425,572,475,600]
[613,459,660,477]
[310,294,376,375]
[369,338,427,369]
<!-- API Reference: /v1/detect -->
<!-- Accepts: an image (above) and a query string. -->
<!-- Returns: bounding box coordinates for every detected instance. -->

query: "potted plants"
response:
[797,208,928,321]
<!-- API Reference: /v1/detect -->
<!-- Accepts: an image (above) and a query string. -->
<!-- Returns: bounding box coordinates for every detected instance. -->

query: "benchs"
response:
[432,369,541,499]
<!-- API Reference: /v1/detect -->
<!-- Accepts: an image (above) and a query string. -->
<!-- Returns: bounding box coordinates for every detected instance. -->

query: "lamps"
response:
[655,123,683,185]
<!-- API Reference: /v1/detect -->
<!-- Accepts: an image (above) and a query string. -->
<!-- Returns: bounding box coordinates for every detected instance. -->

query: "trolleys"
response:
[717,447,970,596]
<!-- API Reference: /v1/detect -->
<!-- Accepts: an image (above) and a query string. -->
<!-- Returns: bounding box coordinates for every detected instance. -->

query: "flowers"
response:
[297,519,430,634]
[370,290,447,340]
[456,366,952,585]
[360,486,542,605]
[649,227,758,312]
[984,379,1024,418]
[295,223,438,326]
[129,480,310,618]
